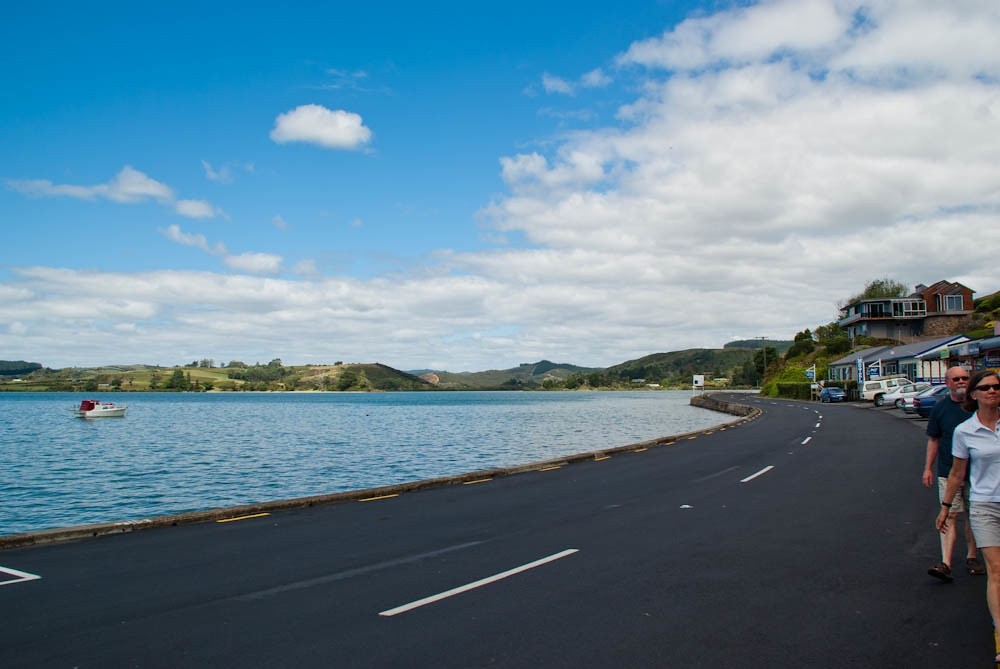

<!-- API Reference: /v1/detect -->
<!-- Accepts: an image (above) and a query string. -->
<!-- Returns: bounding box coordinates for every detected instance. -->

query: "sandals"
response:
[927,562,955,582]
[965,557,986,574]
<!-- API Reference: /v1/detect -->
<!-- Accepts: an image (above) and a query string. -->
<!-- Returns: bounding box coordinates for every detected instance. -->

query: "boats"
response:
[69,400,128,419]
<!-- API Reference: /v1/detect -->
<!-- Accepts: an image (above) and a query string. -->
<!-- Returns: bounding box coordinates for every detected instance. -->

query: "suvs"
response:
[859,378,930,408]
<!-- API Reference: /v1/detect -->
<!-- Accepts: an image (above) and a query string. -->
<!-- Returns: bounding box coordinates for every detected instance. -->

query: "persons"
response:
[933,368,999,664]
[921,365,986,580]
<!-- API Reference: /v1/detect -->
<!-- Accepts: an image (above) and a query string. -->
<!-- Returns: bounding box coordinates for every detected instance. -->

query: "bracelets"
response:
[942,501,953,508]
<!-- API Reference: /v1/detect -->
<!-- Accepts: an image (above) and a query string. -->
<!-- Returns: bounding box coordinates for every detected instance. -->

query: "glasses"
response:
[947,376,970,382]
[974,383,1000,391]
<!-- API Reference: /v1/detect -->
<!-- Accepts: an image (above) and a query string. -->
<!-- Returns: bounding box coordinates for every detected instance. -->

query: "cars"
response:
[881,381,953,417]
[819,387,848,403]
